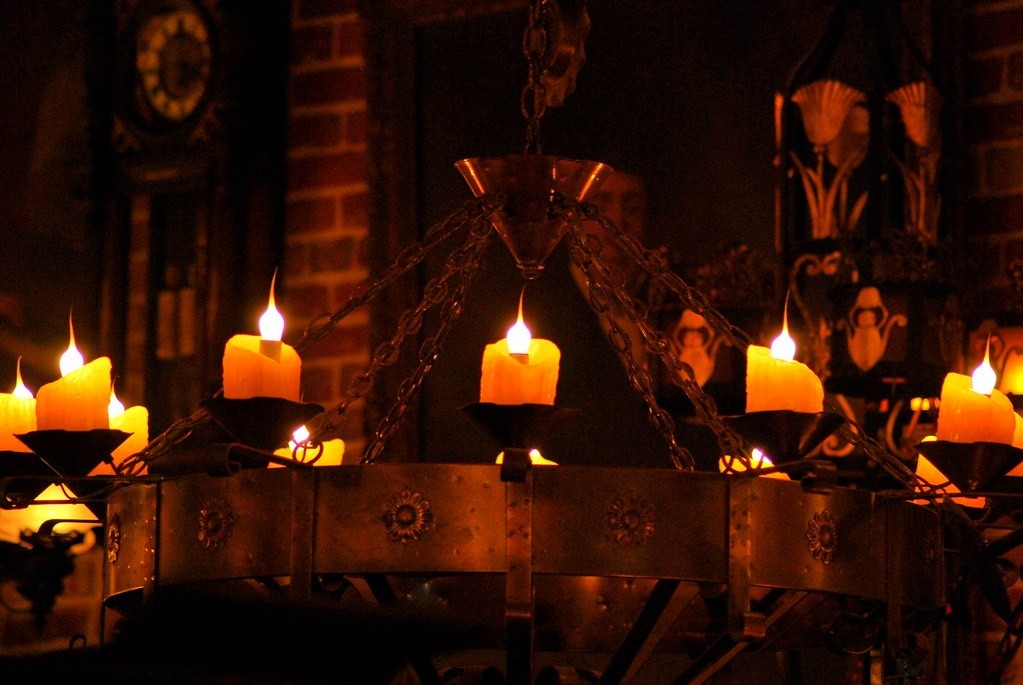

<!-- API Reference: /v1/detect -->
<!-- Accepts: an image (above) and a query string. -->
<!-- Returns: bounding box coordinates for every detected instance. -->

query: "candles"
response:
[0,355,37,454]
[717,444,790,482]
[496,447,556,467]
[221,267,302,405]
[266,425,344,468]
[75,377,151,522]
[38,302,112,431]
[481,287,560,402]
[743,290,823,414]
[912,433,984,509]
[935,338,1013,444]
